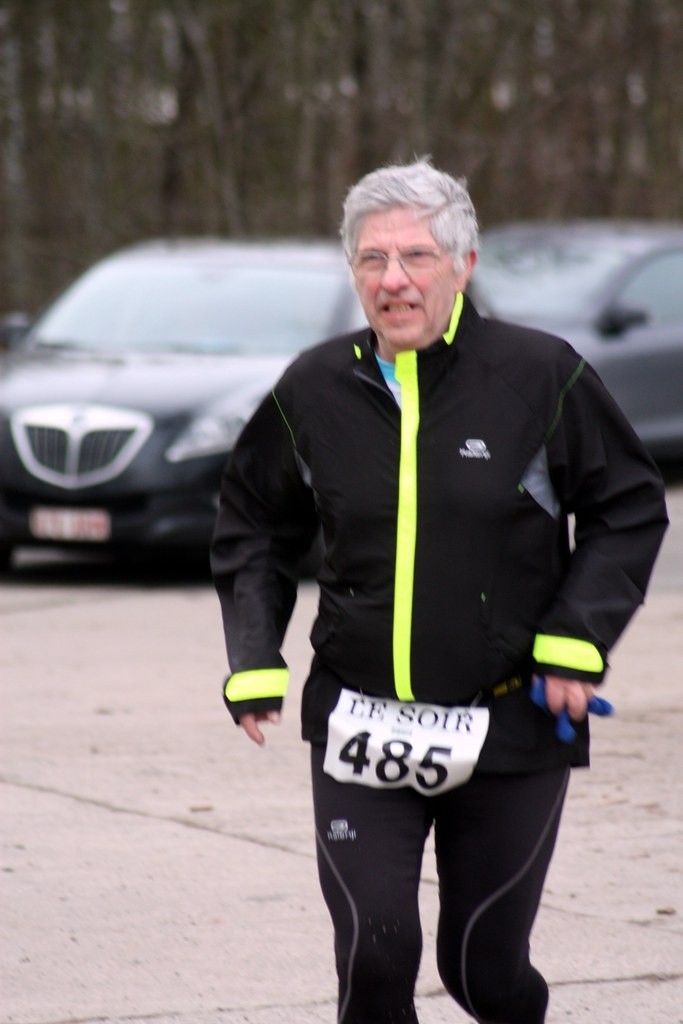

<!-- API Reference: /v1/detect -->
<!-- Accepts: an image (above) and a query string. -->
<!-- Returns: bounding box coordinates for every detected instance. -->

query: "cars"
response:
[0,237,376,580]
[472,212,682,472]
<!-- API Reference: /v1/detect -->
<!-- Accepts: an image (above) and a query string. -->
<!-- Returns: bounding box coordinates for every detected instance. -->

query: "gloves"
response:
[531,678,612,746]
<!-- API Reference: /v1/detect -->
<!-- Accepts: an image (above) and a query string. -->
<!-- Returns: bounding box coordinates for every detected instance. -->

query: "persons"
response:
[208,153,669,1024]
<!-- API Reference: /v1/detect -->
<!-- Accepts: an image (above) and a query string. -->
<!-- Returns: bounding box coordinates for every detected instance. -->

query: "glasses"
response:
[355,245,452,271]
